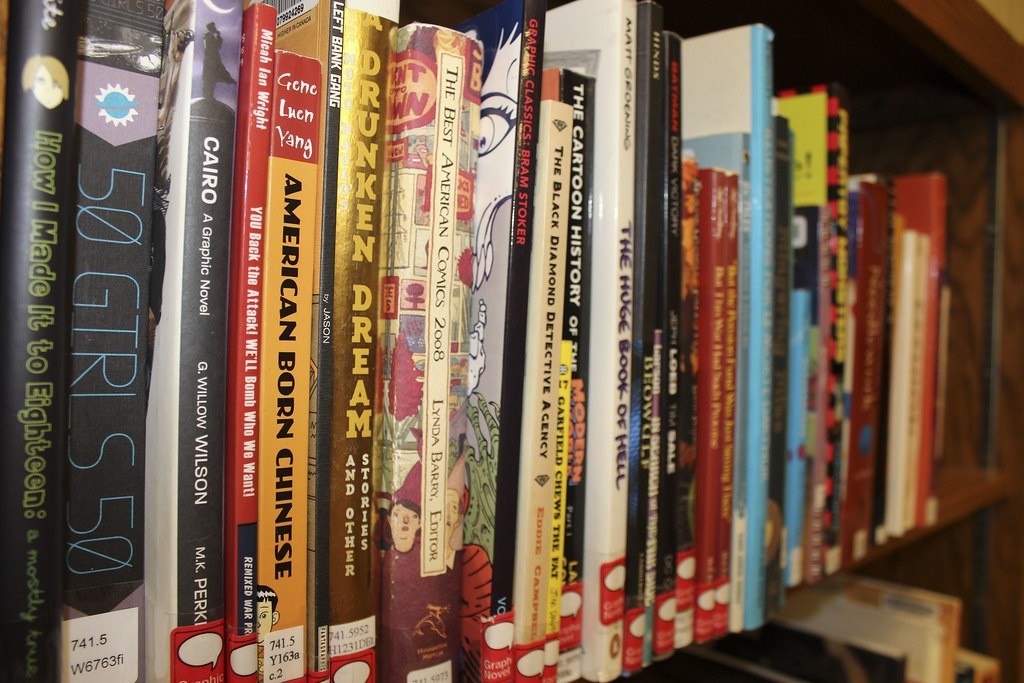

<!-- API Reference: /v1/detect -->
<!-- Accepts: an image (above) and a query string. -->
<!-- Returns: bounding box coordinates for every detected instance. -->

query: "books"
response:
[0,1,1001,683]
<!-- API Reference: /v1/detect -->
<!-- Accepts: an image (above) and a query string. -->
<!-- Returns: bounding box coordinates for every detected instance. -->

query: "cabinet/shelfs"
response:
[0,0,1024,683]
